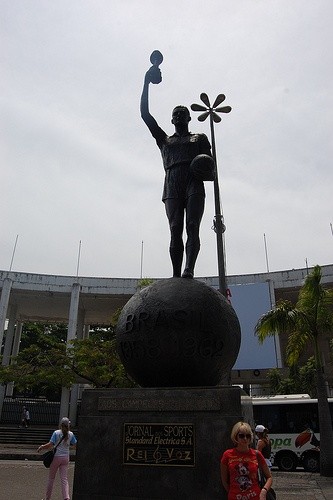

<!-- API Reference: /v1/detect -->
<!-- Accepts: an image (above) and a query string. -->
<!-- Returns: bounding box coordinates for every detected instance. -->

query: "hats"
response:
[59,417,70,426]
[255,425,265,432]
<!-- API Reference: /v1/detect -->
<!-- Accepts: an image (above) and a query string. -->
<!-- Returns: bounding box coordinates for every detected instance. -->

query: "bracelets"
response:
[261,487,267,494]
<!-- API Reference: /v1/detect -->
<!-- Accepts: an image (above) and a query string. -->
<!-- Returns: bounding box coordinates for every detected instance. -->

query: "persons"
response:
[254,424,271,487]
[36,417,76,500]
[17,405,30,428]
[220,421,273,500]
[139,66,217,278]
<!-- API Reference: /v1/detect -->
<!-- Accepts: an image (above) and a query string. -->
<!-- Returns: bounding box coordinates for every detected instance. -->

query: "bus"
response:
[252,395,333,471]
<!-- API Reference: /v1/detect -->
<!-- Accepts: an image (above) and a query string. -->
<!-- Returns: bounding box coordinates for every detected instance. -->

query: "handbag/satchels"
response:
[39,447,56,468]
[259,481,276,500]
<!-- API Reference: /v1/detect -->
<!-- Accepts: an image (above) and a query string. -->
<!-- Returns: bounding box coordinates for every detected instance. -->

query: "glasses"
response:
[238,434,251,439]
